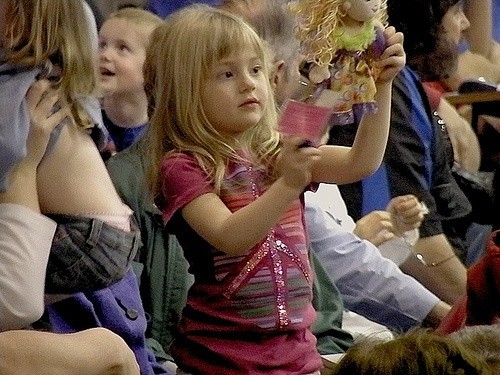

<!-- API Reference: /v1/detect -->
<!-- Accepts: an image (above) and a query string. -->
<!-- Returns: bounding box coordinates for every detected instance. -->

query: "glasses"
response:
[399,205,460,267]
[292,78,316,96]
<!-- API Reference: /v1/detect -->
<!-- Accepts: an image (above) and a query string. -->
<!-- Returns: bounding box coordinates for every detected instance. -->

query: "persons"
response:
[97,7,165,163]
[292,0,499,375]
[1,0,169,375]
[107,0,357,375]
[1,77,143,375]
[142,9,407,375]
